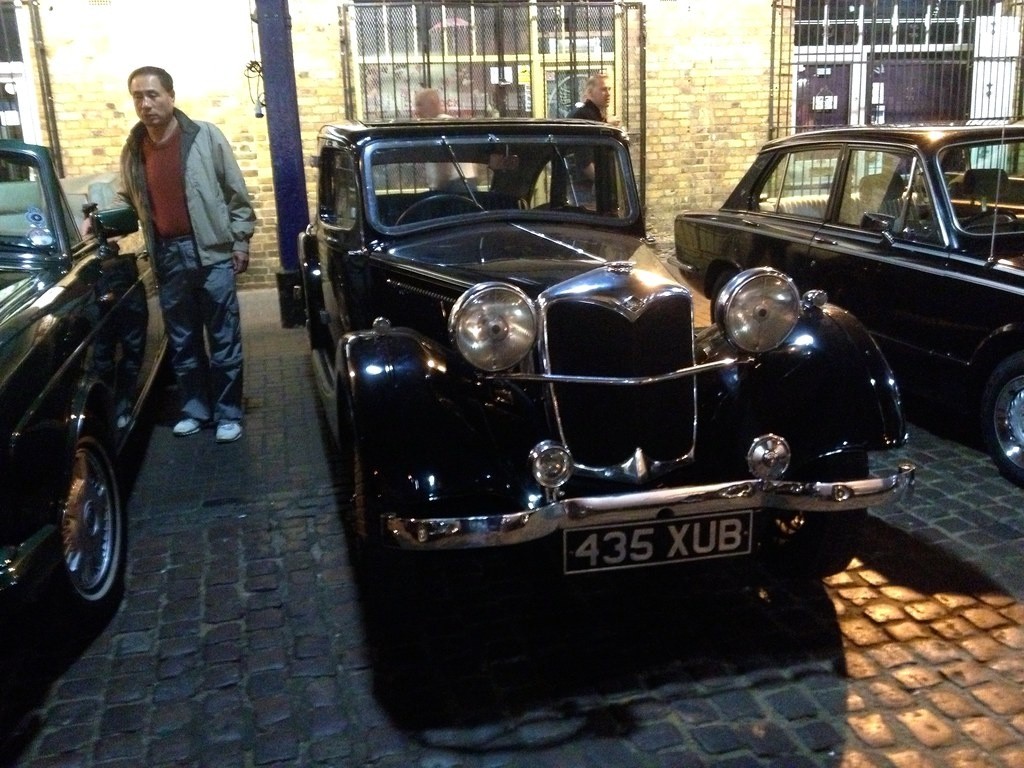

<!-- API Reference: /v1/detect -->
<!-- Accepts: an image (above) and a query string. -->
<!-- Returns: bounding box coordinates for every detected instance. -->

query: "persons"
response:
[80,65,256,443]
[798,104,819,133]
[562,75,620,209]
[415,89,478,191]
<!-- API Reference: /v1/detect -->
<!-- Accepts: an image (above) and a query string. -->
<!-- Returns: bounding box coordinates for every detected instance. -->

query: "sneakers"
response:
[215,423,243,443]
[173,417,200,436]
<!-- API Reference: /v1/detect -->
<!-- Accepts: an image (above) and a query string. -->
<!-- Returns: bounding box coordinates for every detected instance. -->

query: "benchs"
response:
[0,173,126,235]
[376,192,519,227]
[772,171,920,225]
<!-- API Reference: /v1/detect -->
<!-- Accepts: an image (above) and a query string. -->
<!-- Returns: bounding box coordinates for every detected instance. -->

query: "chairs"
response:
[858,171,905,218]
[961,167,1018,224]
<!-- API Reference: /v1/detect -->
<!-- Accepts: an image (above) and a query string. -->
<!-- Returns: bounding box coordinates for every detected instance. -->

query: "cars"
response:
[670,119,1024,484]
[276,124,918,626]
[0,140,172,629]
